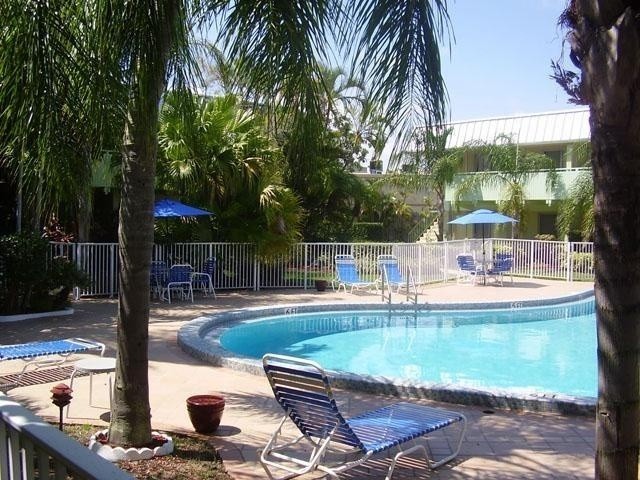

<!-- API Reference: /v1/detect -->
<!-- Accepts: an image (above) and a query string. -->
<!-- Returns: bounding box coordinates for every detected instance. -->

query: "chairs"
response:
[261,352,467,480]
[377,255,426,295]
[332,254,378,297]
[0,336,106,385]
[149,252,218,304]
[455,254,488,288]
[490,250,515,287]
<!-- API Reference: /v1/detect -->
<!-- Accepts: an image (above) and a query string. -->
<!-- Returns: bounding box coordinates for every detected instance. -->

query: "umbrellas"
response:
[448,208,520,267]
[152,200,215,268]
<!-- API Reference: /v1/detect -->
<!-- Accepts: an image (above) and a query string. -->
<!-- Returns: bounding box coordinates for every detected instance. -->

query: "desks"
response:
[64,357,117,419]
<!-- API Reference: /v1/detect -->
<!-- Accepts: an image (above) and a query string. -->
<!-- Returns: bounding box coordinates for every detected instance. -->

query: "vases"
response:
[186,394,225,434]
[315,279,328,291]
